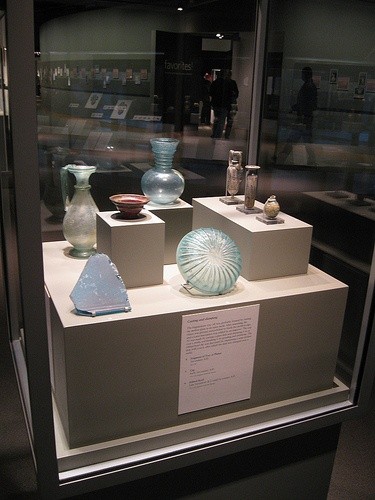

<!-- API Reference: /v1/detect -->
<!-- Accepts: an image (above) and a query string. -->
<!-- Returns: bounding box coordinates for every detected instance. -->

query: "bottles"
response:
[264,195,280,219]
[140,137,185,204]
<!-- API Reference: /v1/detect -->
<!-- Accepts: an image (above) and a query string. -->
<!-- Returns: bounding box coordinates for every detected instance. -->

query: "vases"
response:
[60,163,101,261]
[140,135,189,205]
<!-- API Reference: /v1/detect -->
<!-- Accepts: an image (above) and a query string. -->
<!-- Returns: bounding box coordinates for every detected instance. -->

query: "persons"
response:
[156,51,196,157]
[296,66,318,165]
[200,69,239,140]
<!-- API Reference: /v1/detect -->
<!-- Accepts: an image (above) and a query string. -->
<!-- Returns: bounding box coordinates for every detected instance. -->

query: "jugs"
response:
[43,147,78,223]
[59,163,99,257]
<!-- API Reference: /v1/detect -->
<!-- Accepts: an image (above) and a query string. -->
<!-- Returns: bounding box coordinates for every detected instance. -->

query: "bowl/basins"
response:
[176,228,242,294]
[109,194,151,219]
